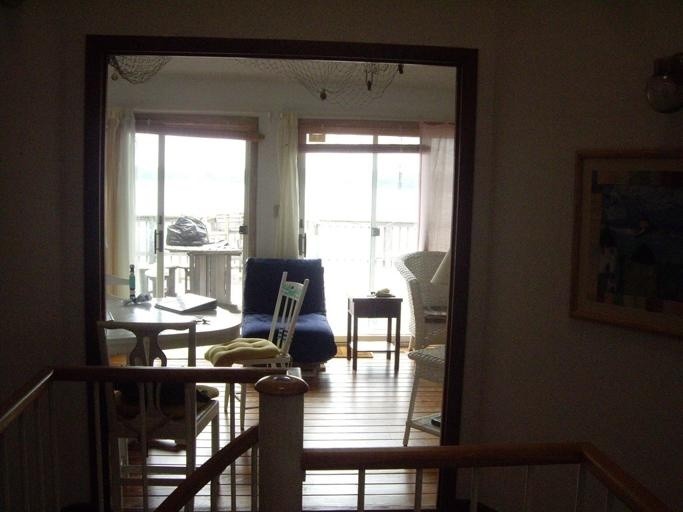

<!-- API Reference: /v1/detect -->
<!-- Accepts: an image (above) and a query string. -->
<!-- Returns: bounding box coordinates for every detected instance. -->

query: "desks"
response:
[105,298,242,366]
[404,346,444,447]
[164,239,242,301]
[347,297,403,373]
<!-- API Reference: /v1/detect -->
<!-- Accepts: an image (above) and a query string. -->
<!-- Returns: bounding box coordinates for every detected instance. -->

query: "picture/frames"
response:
[567,146,683,339]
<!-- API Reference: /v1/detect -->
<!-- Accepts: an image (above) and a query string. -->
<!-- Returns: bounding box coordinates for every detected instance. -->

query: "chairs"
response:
[204,270,309,432]
[96,320,220,512]
[242,257,336,378]
[105,265,135,299]
[396,251,450,353]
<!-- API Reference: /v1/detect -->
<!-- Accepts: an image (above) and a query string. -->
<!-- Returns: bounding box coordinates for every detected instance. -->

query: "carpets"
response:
[334,342,374,358]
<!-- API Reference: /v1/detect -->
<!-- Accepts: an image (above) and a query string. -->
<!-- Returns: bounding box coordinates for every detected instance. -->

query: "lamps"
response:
[645,51,683,113]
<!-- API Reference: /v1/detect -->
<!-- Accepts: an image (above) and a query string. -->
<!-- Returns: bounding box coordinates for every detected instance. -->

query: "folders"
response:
[156,292,218,314]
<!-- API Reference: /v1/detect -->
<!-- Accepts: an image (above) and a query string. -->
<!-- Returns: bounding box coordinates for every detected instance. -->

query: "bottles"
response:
[128,264,135,299]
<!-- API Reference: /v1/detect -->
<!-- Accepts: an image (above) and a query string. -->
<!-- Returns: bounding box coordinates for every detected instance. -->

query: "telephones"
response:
[375,288,394,297]
